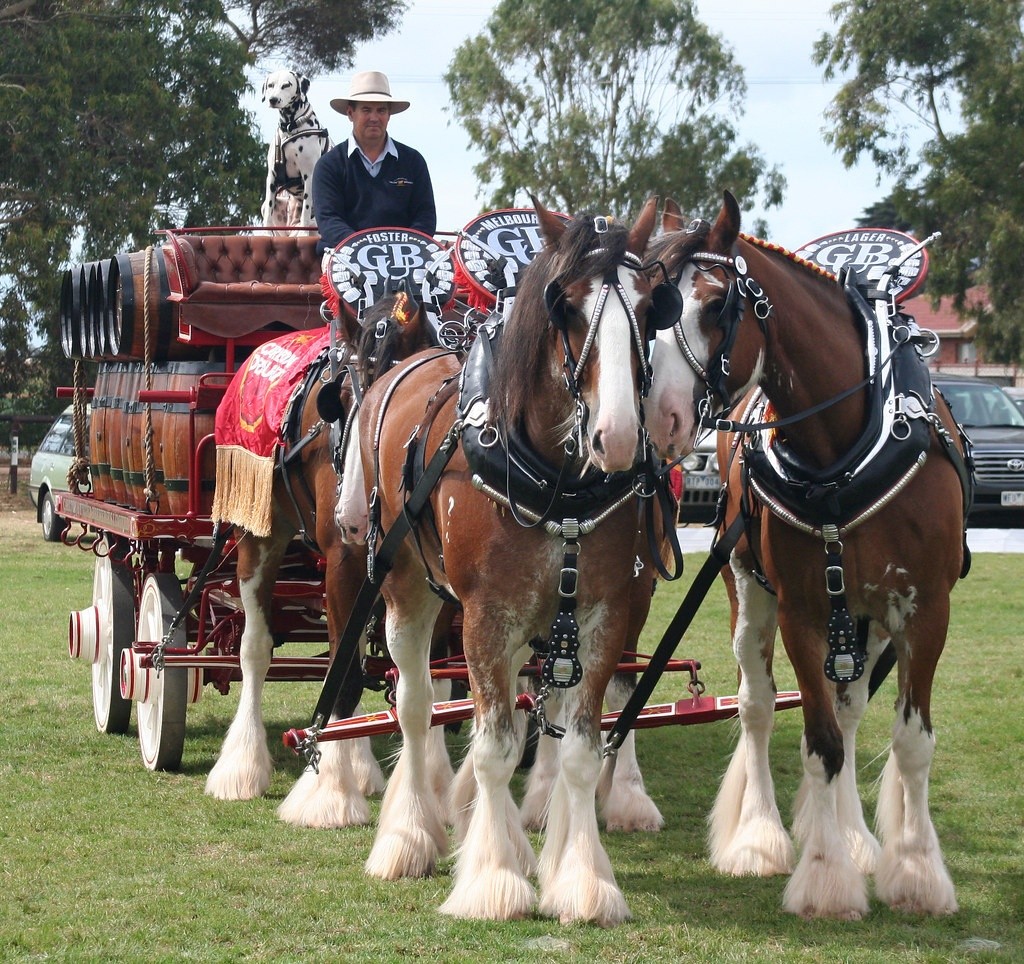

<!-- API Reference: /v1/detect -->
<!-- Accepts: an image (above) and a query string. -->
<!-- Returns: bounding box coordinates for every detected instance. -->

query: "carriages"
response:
[51,171,972,933]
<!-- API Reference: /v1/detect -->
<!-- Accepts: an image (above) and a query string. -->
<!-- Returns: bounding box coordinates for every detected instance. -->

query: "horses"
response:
[203,192,973,925]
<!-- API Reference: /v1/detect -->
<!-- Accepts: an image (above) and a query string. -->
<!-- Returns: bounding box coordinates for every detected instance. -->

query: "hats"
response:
[330,71,410,115]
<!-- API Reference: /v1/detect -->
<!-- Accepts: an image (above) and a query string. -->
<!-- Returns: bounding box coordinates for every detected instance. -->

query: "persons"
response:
[312,71,437,255]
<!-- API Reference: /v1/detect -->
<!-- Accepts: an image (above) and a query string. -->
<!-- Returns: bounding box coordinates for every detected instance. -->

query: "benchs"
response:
[162,230,330,384]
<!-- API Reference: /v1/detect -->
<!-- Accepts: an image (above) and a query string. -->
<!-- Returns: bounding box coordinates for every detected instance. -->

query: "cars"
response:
[928,372,1024,508]
[681,426,721,510]
[30,403,93,538]
[960,385,1024,425]
